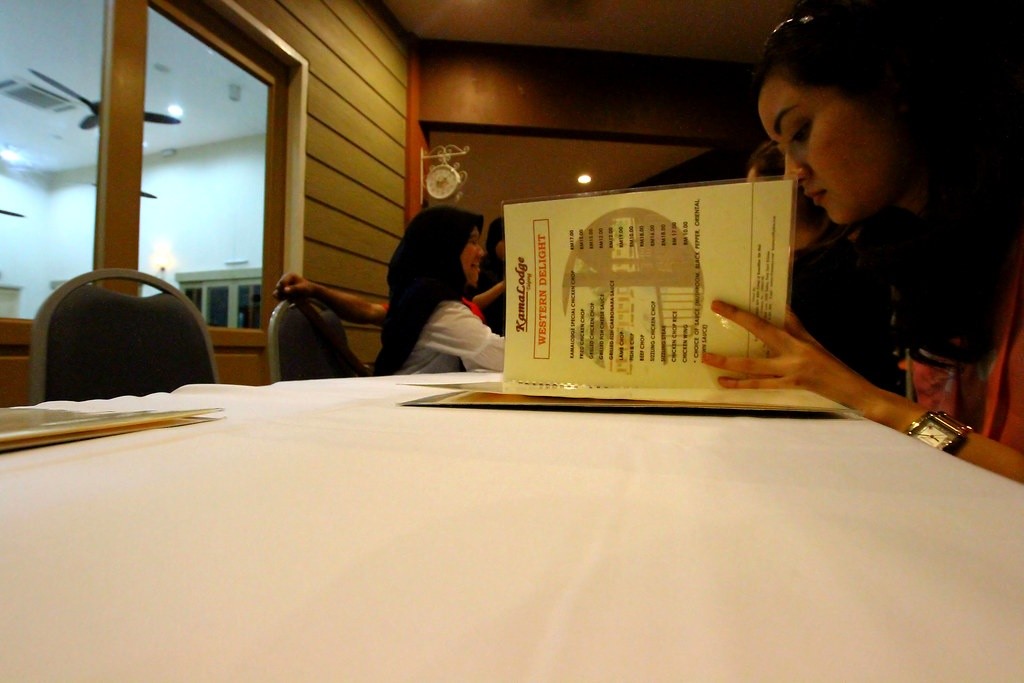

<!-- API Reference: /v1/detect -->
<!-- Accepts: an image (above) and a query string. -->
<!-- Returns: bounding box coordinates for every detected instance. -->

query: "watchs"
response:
[904,411,973,451]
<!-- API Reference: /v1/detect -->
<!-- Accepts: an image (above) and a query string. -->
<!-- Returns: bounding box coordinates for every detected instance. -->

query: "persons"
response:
[701,0,1024,485]
[272,204,505,377]
[239,294,260,328]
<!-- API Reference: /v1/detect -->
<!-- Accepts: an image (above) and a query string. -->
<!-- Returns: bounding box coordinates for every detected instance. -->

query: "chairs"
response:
[266,296,364,387]
[30,267,219,411]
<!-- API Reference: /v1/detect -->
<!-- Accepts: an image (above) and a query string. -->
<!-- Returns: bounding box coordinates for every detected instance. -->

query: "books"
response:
[400,174,864,415]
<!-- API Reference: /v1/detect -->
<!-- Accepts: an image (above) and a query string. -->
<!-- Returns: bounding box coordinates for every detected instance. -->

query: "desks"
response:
[0,367,1024,683]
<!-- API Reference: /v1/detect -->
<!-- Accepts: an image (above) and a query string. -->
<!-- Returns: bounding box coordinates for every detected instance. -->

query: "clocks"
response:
[421,143,471,207]
